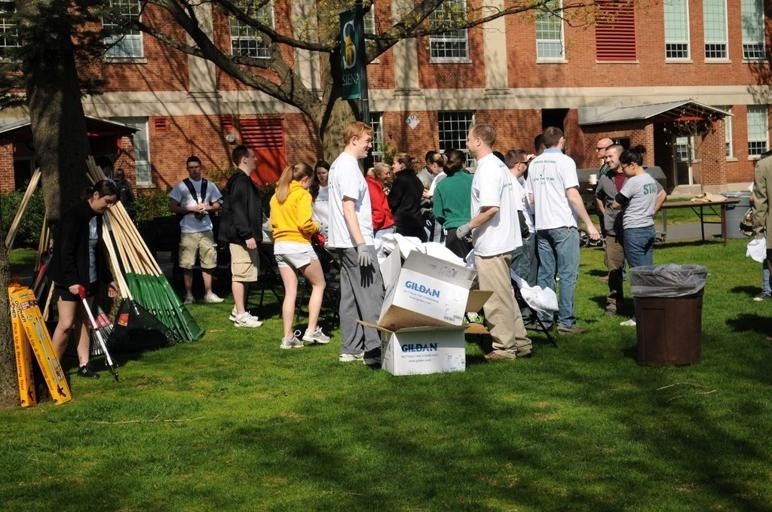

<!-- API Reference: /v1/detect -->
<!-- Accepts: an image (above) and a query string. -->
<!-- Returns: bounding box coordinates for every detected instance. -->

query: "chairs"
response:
[243,241,283,322]
[298,235,343,335]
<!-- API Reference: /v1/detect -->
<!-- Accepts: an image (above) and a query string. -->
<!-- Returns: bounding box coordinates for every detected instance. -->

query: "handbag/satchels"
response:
[739,208,755,236]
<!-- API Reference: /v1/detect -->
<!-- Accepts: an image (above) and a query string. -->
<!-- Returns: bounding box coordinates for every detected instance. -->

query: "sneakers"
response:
[603,308,615,318]
[204,292,225,303]
[339,347,381,366]
[557,323,588,335]
[183,292,194,306]
[480,343,532,361]
[620,317,636,328]
[78,364,100,381]
[229,304,264,328]
[303,328,330,344]
[280,334,304,349]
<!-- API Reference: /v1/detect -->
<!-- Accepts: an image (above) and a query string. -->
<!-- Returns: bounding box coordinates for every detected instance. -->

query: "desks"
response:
[659,198,741,245]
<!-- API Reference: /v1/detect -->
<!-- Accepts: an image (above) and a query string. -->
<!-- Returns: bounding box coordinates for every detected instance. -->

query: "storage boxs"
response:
[375,247,478,331]
[355,318,488,377]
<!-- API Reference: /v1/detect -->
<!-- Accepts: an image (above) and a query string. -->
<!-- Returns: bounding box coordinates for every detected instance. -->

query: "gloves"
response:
[320,222,329,239]
[455,223,471,241]
[356,244,375,267]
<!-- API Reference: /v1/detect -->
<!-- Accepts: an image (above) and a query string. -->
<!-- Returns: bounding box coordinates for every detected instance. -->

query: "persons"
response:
[326,121,389,365]
[48,180,119,378]
[739,150,771,303]
[748,155,771,289]
[115,168,134,220]
[266,163,332,348]
[167,156,226,305]
[456,122,533,359]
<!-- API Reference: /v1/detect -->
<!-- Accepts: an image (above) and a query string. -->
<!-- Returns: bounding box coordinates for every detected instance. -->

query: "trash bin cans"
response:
[720,191,753,240]
[629,264,708,367]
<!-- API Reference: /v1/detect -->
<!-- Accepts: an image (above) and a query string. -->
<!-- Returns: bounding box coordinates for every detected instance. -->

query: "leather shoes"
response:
[753,289,771,301]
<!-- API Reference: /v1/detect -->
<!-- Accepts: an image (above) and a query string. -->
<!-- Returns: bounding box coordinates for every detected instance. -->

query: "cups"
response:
[589,173,597,186]
